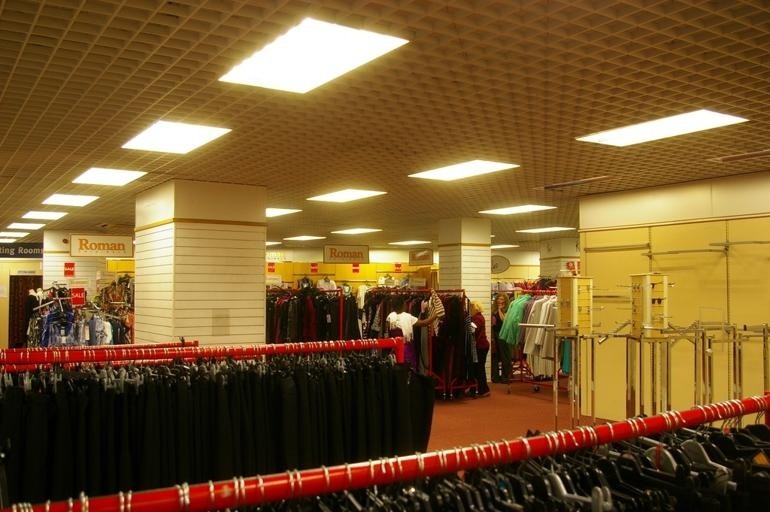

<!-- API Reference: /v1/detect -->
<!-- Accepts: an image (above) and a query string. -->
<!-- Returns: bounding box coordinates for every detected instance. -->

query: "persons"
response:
[491,293,512,382]
[469,301,491,396]
[387,297,437,374]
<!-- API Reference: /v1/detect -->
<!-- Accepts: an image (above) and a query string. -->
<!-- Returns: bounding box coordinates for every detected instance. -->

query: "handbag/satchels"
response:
[471,340,479,363]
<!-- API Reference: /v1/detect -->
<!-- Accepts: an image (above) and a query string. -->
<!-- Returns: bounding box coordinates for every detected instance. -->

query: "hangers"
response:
[491,279,559,303]
[26,273,136,332]
[268,269,470,309]
